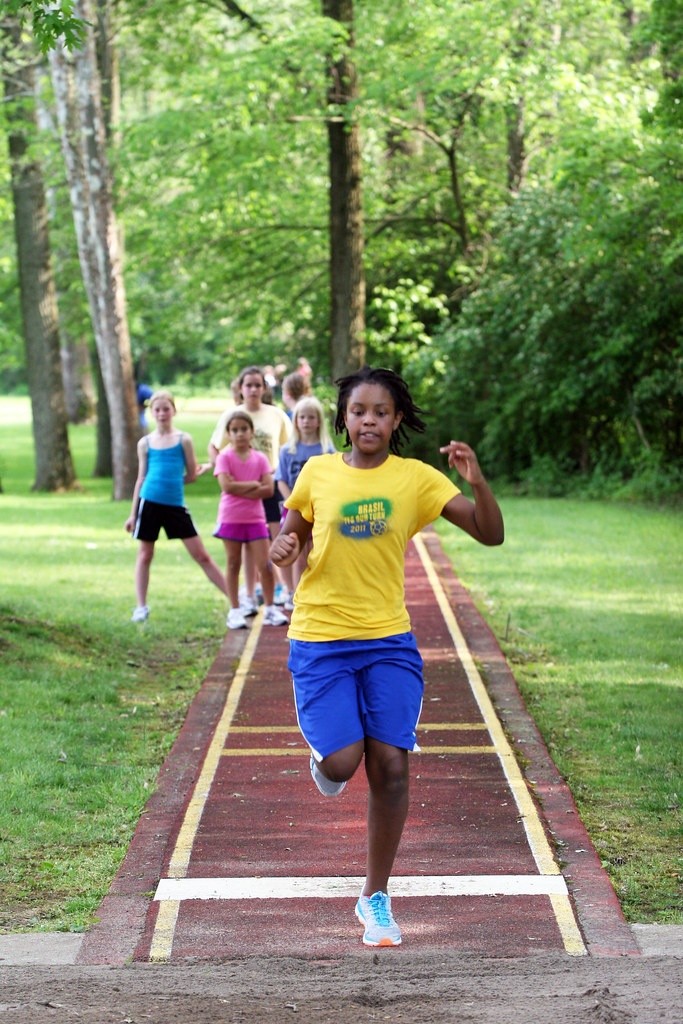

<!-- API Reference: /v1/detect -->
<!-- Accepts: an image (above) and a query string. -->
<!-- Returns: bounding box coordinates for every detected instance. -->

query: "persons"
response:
[206,367,288,605]
[213,410,289,629]
[124,390,258,624]
[296,358,313,378]
[271,366,503,948]
[136,379,154,431]
[281,373,310,427]
[275,399,335,610]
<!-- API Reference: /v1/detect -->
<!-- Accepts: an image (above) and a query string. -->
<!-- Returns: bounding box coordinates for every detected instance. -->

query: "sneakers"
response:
[309,754,346,797]
[355,891,402,946]
[131,606,149,624]
[226,583,295,629]
